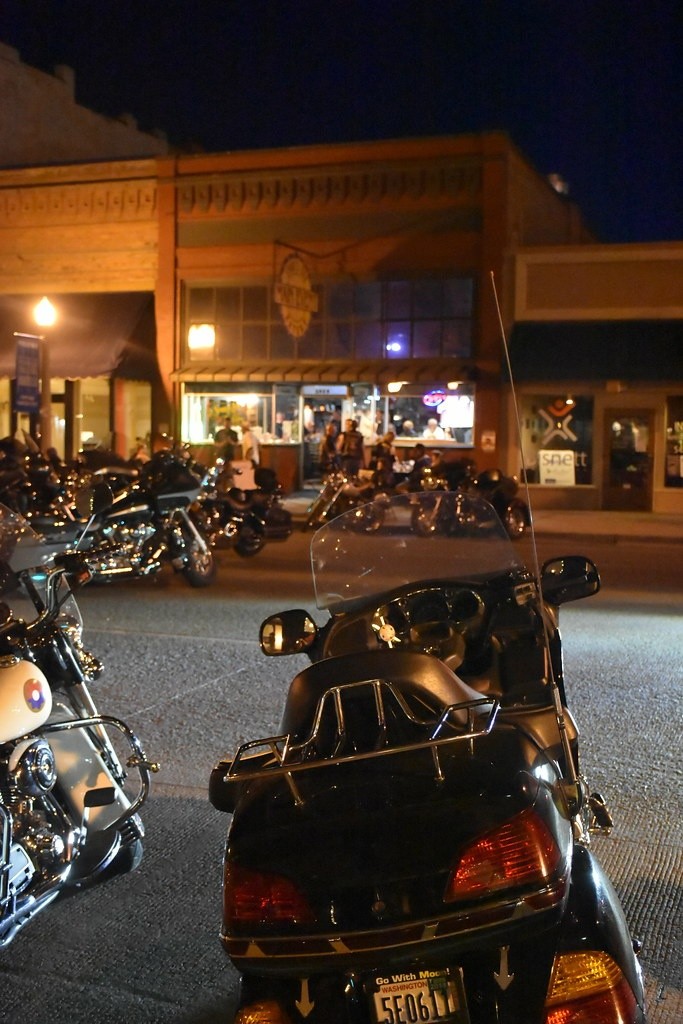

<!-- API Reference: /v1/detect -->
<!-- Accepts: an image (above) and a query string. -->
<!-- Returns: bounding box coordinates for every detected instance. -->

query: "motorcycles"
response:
[1,503,162,952]
[0,427,532,597]
[204,484,651,1023]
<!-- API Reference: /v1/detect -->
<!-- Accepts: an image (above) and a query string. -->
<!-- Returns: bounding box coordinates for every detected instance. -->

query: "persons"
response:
[216,418,263,484]
[274,396,470,493]
[130,437,147,464]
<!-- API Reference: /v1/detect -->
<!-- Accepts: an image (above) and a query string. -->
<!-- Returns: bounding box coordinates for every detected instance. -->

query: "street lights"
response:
[31,295,59,458]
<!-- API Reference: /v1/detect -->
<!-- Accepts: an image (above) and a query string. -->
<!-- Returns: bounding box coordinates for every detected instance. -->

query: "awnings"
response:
[170,359,468,384]
